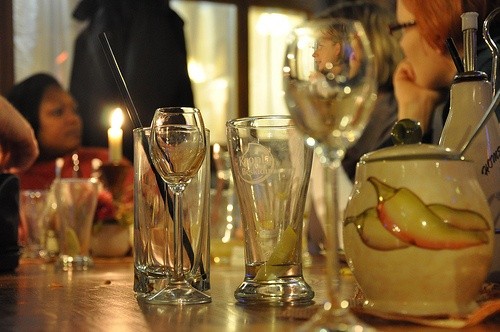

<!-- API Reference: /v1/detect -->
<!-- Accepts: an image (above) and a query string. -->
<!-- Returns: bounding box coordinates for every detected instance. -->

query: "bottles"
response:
[437,11,500,282]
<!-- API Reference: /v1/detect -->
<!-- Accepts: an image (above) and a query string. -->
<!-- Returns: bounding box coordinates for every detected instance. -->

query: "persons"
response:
[0,72,161,245]
[393,0,500,149]
[312,0,402,185]
[69,0,196,165]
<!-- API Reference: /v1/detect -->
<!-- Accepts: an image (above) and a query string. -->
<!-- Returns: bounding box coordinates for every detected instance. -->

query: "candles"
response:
[107,107,125,165]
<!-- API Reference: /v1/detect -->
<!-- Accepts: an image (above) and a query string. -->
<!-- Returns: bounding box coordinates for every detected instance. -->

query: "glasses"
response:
[388,22,415,38]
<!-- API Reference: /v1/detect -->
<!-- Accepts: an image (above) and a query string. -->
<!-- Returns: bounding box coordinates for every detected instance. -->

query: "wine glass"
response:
[146,107,212,306]
[281,17,378,332]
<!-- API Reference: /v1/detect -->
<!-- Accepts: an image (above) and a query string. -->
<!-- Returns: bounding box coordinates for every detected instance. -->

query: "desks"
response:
[0,236,500,332]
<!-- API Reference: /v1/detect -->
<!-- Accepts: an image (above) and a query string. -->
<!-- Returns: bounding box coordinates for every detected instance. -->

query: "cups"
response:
[224,114,313,309]
[18,190,57,272]
[131,124,211,293]
[53,178,99,273]
[344,118,494,318]
